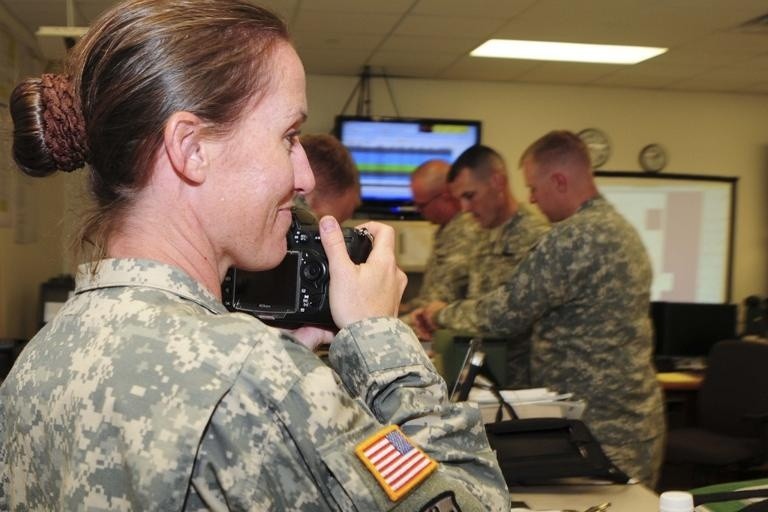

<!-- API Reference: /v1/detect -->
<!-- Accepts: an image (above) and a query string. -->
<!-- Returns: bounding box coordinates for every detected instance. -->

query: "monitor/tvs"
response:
[335,114,481,221]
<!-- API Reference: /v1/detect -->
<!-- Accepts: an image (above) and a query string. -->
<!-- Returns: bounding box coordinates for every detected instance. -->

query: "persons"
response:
[398,158,479,355]
[417,128,667,491]
[407,143,550,390]
[0,0,512,512]
[290,130,362,352]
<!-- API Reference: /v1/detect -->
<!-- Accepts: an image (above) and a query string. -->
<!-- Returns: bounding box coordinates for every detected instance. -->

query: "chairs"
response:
[662,340,768,480]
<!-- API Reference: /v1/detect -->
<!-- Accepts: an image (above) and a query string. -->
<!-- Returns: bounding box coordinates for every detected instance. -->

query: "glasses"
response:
[409,191,449,210]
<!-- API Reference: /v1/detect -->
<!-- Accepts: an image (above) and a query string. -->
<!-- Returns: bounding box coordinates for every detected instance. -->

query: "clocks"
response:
[639,143,668,172]
[577,128,611,168]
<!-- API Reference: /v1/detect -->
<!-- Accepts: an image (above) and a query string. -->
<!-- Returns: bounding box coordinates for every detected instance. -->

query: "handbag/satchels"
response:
[483,417,634,488]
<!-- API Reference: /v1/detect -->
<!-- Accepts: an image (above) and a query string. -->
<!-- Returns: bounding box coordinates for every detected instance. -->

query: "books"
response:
[688,477,768,512]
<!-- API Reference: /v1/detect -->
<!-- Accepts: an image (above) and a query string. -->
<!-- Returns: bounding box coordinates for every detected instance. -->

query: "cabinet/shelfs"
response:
[341,219,443,311]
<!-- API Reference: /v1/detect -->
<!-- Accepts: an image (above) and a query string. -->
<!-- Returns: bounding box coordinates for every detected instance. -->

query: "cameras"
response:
[220,206,372,335]
